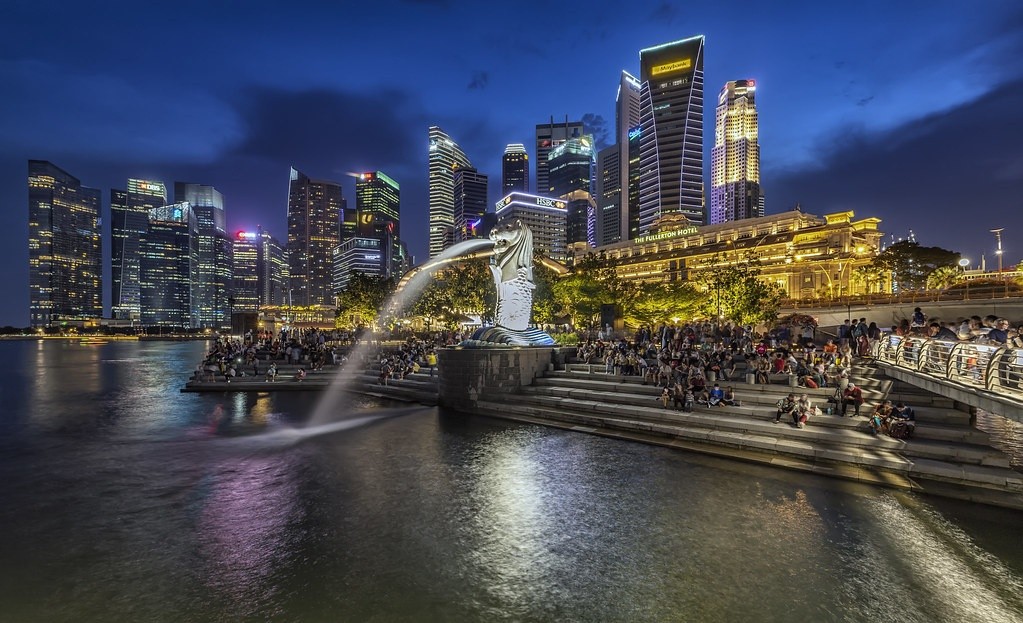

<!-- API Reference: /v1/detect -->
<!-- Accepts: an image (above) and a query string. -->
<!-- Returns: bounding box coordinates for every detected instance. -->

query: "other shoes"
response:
[709,402,715,406]
[656,384,661,387]
[798,385,806,388]
[642,381,648,385]
[854,414,859,416]
[842,412,846,416]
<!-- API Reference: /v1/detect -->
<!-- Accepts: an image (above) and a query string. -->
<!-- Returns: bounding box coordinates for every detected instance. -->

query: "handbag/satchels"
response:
[759,374,767,384]
[814,405,823,416]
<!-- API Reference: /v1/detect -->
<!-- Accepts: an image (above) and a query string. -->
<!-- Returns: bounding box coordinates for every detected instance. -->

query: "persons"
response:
[196,326,462,386]
[891,305,1023,390]
[576,318,915,439]
[546,324,572,334]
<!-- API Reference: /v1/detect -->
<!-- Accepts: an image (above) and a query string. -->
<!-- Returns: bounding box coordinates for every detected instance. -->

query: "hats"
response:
[848,383,855,387]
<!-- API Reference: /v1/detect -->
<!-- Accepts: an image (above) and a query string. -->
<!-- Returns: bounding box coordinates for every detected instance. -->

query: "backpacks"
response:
[915,312,924,324]
